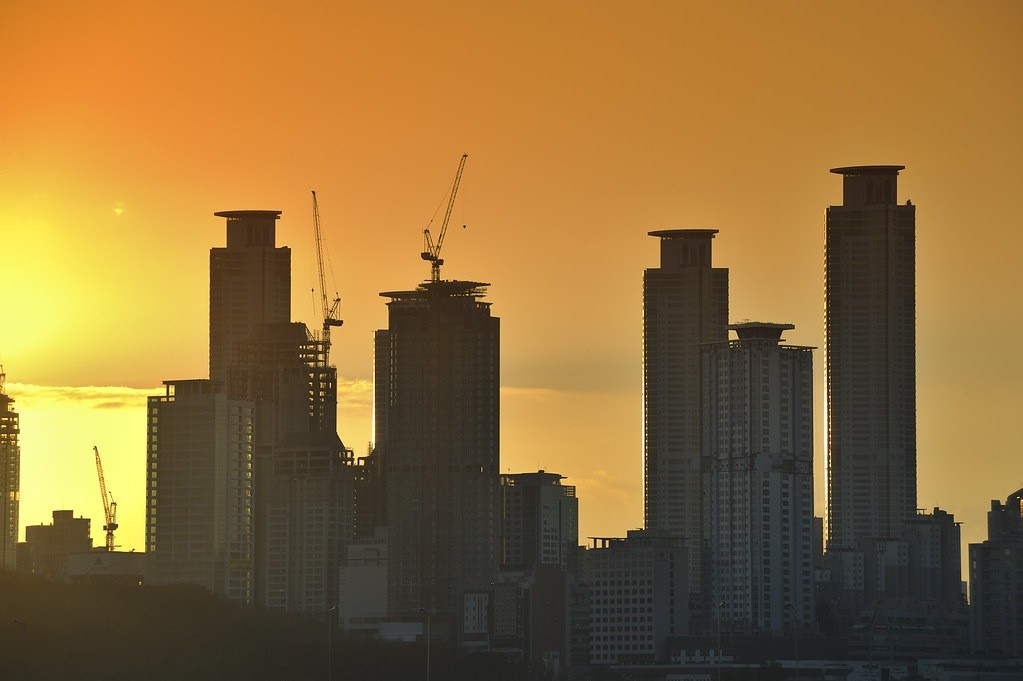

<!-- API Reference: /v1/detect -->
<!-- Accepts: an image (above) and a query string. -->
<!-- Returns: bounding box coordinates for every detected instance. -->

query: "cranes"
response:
[420,153,468,285]
[91,445,122,552]
[309,189,345,405]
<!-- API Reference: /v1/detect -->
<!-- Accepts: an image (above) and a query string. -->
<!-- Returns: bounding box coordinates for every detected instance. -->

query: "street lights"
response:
[785,602,799,681]
[417,607,431,681]
[715,603,723,681]
